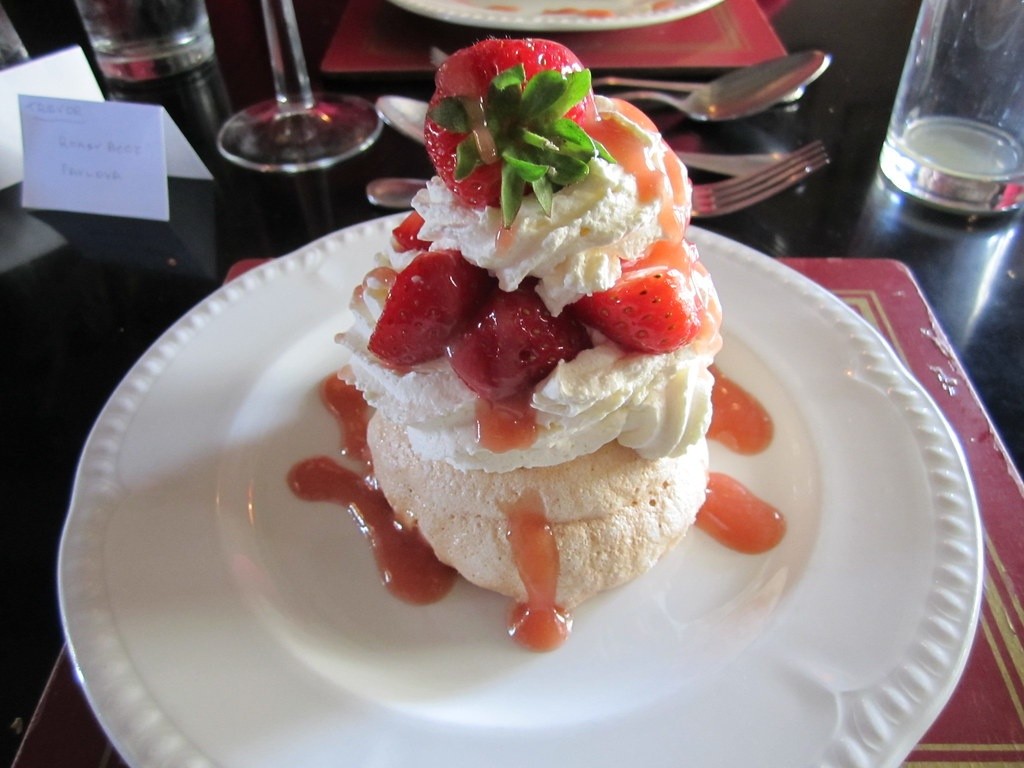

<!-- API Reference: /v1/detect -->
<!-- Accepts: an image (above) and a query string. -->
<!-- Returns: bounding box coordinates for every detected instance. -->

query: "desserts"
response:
[334,38,722,608]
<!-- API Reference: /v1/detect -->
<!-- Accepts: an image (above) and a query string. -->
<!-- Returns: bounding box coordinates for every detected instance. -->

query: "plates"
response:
[56,210,984,768]
[387,0,724,31]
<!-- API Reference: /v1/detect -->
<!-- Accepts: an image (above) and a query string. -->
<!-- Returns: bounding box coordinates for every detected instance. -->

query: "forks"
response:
[366,139,829,217]
[431,46,806,102]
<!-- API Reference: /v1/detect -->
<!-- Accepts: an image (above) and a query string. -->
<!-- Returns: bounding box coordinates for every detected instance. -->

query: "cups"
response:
[879,0,1024,217]
[74,0,216,81]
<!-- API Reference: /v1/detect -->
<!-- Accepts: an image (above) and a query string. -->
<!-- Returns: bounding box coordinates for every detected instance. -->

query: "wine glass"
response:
[216,0,384,173]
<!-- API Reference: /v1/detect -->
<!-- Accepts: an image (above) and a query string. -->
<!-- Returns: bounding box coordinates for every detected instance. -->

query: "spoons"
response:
[374,95,789,178]
[606,49,831,120]
